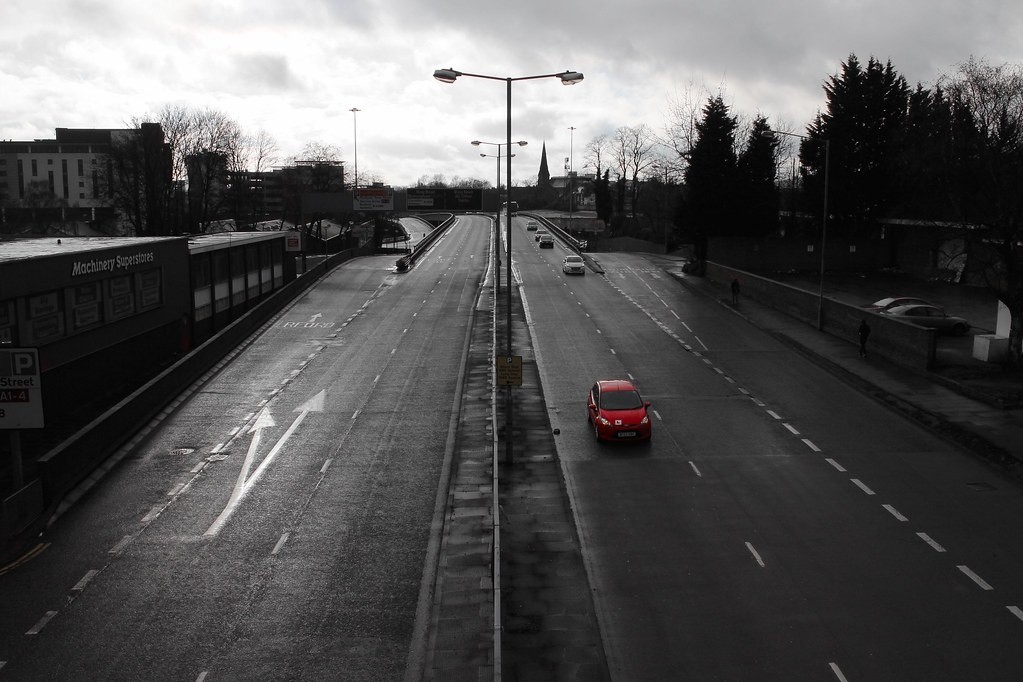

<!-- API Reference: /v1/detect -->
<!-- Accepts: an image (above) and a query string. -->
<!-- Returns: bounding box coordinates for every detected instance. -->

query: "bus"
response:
[503,201,519,217]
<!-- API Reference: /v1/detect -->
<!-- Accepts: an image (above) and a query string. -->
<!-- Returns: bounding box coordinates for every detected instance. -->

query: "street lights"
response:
[350,108,362,195]
[761,130,831,333]
[432,67,584,468]
[567,126,576,236]
[478,154,516,189]
[470,141,528,293]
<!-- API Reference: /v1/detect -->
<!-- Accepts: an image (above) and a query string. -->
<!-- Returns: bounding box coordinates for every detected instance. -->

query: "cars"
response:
[561,255,586,275]
[539,234,554,248]
[870,297,946,313]
[587,380,653,445]
[534,230,548,241]
[526,221,537,231]
[879,304,971,336]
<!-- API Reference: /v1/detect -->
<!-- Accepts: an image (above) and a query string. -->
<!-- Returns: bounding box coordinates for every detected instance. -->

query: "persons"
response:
[857,320,871,358]
[731,279,741,303]
